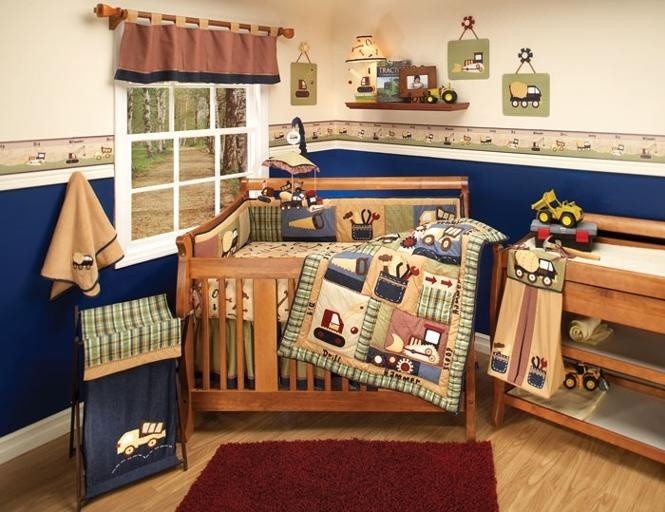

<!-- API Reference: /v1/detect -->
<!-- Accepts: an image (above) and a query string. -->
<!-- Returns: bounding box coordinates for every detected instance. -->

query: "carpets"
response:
[177,441,497,509]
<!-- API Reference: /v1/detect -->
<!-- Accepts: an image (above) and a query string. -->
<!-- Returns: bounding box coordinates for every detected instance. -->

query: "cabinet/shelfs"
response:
[490,196,664,467]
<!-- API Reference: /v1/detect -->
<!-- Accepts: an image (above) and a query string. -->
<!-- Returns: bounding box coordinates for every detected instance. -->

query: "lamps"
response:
[346,36,386,63]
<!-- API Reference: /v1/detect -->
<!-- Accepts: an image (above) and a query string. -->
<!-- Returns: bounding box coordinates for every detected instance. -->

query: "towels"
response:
[40,172,124,301]
[568,314,614,348]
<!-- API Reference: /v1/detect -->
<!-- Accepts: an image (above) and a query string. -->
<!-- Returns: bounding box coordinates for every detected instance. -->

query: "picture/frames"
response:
[398,66,438,99]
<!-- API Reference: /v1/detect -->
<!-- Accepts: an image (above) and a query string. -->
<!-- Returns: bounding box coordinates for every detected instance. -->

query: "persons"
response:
[407,74,428,90]
